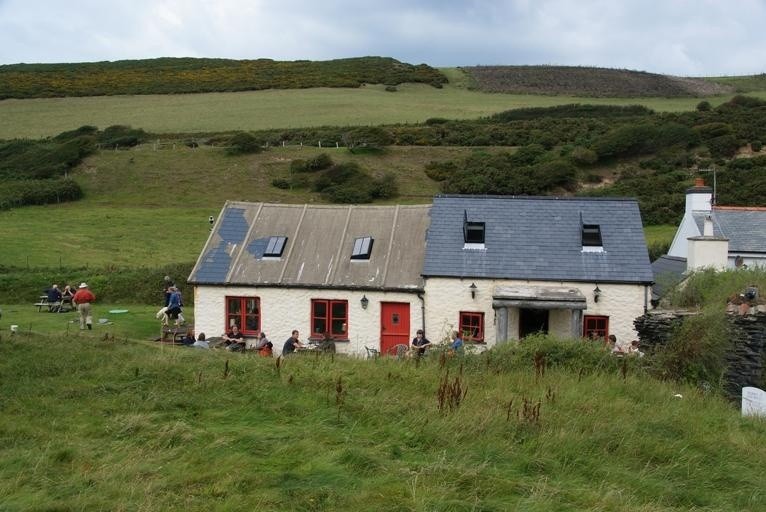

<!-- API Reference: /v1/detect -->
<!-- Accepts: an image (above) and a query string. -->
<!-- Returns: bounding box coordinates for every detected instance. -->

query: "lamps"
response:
[592,284,602,303]
[469,282,478,300]
[360,295,368,309]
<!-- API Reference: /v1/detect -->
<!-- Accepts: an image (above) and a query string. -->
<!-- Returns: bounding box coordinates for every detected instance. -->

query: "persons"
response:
[183,330,195,347]
[43,282,61,309]
[221,324,245,354]
[282,329,305,355]
[61,285,73,297]
[314,332,336,356]
[604,336,623,351]
[445,329,464,349]
[254,331,274,357]
[410,329,432,357]
[155,274,184,326]
[73,279,97,333]
[190,333,211,349]
[627,340,645,356]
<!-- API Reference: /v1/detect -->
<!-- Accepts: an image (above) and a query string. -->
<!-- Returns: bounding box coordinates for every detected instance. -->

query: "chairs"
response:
[364,344,408,365]
[226,339,245,352]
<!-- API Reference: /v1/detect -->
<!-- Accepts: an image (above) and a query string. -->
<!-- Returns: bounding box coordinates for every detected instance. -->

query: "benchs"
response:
[167,342,184,345]
[33,302,59,306]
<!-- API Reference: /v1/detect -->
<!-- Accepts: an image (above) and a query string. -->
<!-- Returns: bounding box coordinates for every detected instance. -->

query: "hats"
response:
[78,283,88,288]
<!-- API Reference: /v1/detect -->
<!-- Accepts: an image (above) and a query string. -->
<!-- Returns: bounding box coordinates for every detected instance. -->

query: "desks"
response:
[243,348,261,353]
[296,342,329,356]
[204,336,227,350]
[177,334,187,341]
[38,295,71,313]
